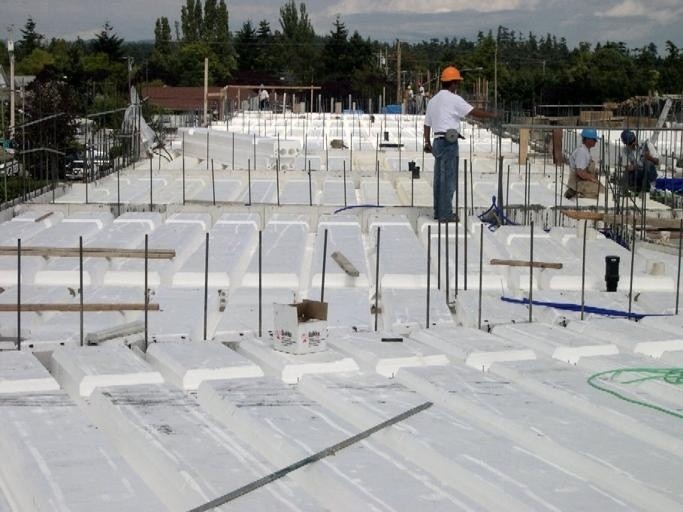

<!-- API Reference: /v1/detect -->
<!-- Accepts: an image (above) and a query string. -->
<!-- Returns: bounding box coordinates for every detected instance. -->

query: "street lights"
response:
[387,70,406,81]
[419,67,483,92]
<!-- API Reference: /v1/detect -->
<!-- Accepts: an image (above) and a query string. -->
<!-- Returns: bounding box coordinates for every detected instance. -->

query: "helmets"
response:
[438,64,465,84]
[579,127,601,141]
[620,129,637,143]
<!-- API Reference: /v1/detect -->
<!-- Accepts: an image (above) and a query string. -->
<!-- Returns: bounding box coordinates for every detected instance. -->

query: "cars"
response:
[0,148,18,178]
[64,151,114,182]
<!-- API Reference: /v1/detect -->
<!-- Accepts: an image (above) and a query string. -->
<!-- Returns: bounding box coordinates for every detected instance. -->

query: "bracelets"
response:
[424,140,430,146]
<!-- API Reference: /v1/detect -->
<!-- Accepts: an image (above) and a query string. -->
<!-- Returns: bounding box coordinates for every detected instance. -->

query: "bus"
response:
[67,119,96,145]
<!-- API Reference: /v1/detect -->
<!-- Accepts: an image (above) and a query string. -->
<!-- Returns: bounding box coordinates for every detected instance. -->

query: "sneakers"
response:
[433,210,460,223]
[565,187,582,198]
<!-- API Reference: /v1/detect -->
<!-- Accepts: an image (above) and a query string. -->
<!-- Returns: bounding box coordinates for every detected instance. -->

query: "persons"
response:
[561,124,608,202]
[618,128,659,196]
[406,83,425,114]
[422,65,506,224]
